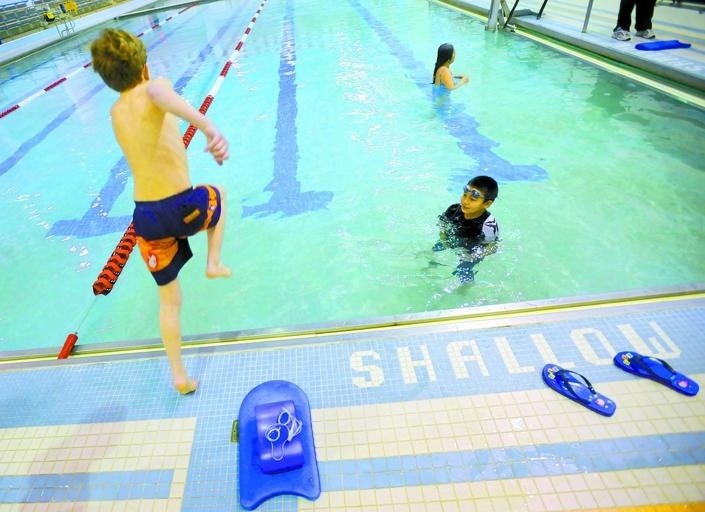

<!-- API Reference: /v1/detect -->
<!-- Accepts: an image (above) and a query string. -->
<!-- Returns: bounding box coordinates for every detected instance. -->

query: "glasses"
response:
[463,186,487,202]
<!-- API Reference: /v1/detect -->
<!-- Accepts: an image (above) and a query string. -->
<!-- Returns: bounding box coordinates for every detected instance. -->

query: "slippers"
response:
[542,362,617,419]
[614,351,701,398]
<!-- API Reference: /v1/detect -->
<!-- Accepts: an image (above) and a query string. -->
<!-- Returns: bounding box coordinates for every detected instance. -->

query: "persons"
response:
[89,30,232,394]
[434,176,500,285]
[613,1,657,41]
[433,43,467,92]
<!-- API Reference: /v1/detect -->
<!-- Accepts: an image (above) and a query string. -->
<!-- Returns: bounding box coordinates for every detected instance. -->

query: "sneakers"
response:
[612,26,655,41]
